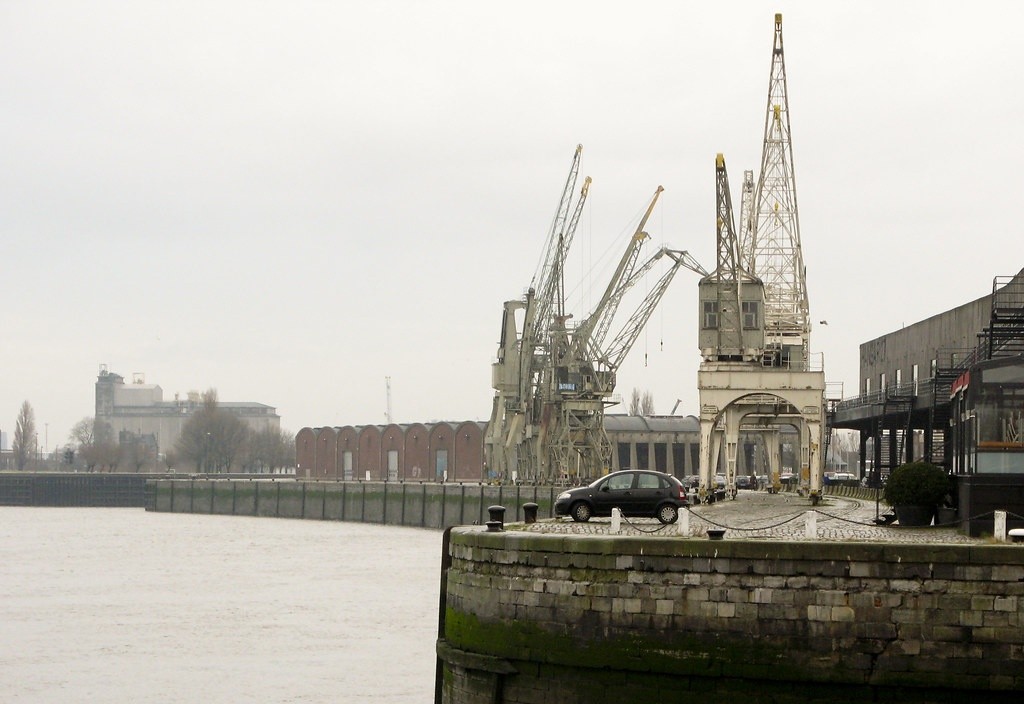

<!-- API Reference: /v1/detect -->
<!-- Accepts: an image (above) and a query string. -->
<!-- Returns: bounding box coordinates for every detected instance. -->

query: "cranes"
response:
[484,143,712,484]
[698,28,826,497]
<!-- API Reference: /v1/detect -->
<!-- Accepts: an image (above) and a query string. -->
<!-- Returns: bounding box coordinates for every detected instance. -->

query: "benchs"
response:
[715,490,727,502]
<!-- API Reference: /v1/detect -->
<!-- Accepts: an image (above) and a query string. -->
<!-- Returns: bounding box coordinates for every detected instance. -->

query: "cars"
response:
[555,470,689,524]
[683,471,858,490]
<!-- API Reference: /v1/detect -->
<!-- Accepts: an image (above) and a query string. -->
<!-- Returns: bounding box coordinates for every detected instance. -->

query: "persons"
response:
[750,474,756,491]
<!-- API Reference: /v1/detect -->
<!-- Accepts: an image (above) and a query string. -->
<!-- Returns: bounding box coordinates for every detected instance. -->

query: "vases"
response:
[934,507,956,527]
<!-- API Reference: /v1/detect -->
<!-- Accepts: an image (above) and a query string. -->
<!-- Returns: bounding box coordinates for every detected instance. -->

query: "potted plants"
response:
[884,461,951,527]
